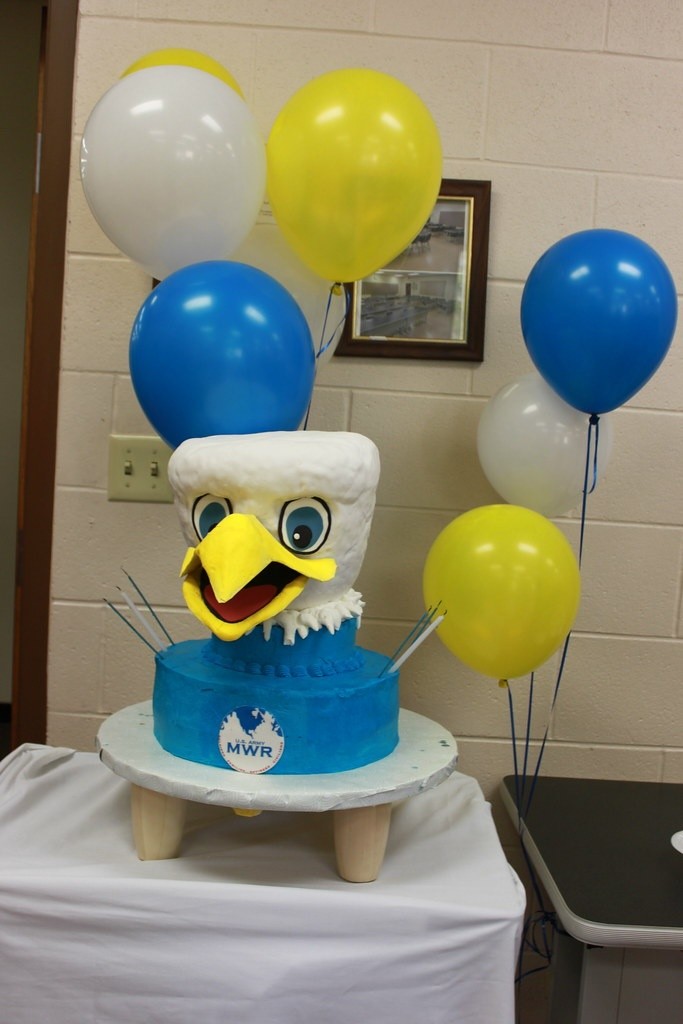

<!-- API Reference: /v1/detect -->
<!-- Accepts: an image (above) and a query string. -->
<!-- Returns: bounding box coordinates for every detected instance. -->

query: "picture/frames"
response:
[328,179,490,361]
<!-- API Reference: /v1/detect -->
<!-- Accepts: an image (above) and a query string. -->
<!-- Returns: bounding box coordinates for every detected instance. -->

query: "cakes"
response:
[148,432,402,775]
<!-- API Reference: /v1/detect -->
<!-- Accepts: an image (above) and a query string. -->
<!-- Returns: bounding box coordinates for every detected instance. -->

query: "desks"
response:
[501,772,683,1024]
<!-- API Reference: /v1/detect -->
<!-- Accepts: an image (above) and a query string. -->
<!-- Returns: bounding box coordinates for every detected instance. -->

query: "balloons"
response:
[77,68,268,282]
[422,505,585,687]
[268,69,445,287]
[116,49,244,97]
[478,376,609,514]
[129,260,315,460]
[521,229,678,418]
[223,226,349,365]
